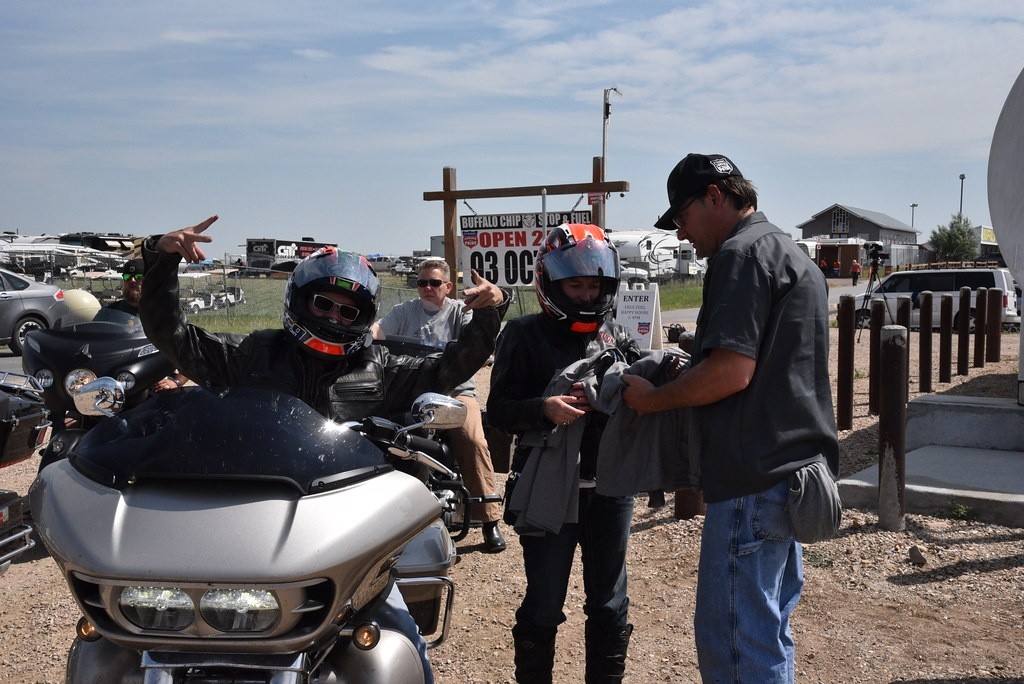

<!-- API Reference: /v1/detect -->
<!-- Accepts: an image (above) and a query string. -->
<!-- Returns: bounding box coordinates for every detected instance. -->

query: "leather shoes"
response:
[482,520,506,550]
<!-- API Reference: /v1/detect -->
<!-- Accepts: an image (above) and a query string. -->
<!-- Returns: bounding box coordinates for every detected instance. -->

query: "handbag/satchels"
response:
[504,475,523,526]
[785,461,842,542]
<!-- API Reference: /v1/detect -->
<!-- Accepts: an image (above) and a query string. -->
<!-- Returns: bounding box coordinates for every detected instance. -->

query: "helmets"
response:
[535,224,621,333]
[282,246,382,355]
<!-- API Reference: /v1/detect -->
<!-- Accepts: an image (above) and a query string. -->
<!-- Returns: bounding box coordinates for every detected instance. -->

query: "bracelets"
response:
[167,377,181,387]
[544,396,551,408]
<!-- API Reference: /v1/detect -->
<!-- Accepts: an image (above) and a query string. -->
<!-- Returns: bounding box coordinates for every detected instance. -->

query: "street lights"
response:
[957,173,967,218]
[909,203,919,228]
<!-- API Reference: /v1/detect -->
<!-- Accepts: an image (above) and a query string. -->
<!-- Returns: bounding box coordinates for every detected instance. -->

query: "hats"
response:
[654,153,742,230]
[122,259,146,276]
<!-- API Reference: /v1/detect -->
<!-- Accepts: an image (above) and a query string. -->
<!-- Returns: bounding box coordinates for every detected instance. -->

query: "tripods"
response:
[853,259,895,344]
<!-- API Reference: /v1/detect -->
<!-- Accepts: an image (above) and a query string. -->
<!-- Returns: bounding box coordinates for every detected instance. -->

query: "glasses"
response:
[309,293,363,322]
[672,193,703,229]
[417,278,448,288]
[123,273,145,283]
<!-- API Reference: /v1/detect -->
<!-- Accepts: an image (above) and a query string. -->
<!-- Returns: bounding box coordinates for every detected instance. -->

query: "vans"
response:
[852,268,1019,333]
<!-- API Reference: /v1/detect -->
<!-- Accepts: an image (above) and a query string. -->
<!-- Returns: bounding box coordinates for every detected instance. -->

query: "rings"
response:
[562,419,571,425]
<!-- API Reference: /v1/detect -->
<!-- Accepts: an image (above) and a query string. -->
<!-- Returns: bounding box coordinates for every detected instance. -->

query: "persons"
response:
[234,258,244,266]
[91,258,190,391]
[820,256,827,275]
[849,260,863,287]
[621,153,840,684]
[834,258,841,278]
[369,257,507,552]
[486,223,643,684]
[140,216,512,684]
[868,259,874,277]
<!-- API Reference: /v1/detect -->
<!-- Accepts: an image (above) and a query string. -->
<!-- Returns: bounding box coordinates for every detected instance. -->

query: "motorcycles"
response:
[18,368,458,682]
[333,336,504,543]
[15,305,177,463]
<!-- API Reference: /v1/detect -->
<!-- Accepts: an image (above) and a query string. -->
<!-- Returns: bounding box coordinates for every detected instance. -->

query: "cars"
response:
[367,256,413,276]
[0,267,67,357]
[620,265,648,286]
